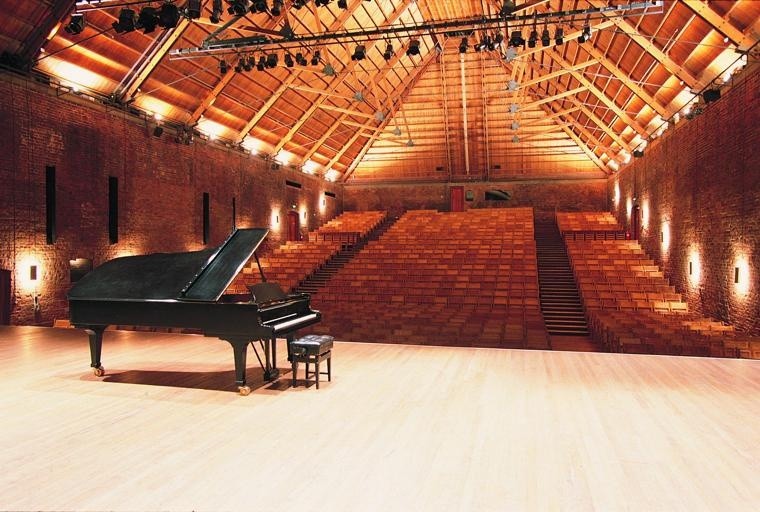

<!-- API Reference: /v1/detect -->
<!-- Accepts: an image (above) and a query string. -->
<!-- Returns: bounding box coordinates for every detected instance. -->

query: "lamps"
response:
[458,23,593,53]
[63,0,348,36]
[218,36,420,78]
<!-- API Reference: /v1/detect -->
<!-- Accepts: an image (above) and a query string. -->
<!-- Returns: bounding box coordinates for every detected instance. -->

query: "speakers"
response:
[634,150,644,158]
[153,126,163,138]
[703,89,721,104]
[270,163,279,170]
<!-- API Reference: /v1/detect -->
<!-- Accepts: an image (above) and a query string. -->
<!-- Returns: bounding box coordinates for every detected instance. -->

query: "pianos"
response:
[68,229,321,396]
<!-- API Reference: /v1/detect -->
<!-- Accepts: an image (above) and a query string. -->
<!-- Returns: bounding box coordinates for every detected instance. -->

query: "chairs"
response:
[223,206,760,358]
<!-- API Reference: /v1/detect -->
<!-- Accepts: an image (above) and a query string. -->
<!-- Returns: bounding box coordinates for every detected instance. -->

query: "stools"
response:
[289,334,336,389]
